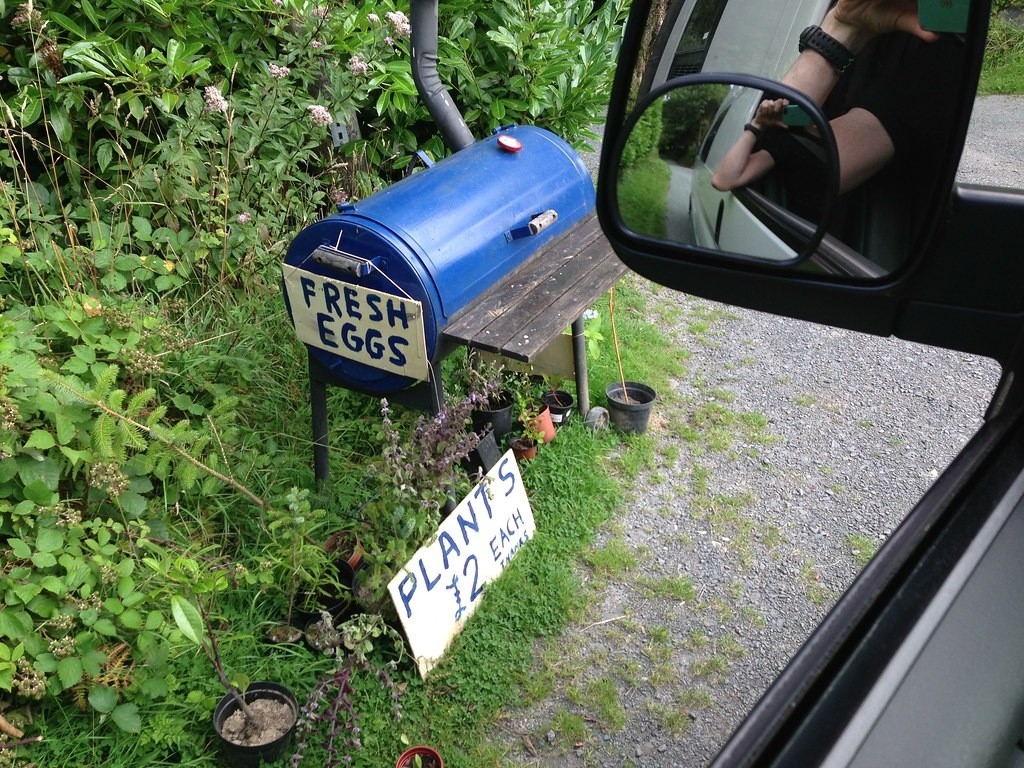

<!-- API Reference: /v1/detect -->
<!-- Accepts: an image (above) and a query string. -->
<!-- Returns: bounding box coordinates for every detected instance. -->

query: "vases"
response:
[604,381,655,434]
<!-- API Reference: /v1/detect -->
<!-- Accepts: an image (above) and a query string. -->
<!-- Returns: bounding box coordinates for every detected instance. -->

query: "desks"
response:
[450,213,632,415]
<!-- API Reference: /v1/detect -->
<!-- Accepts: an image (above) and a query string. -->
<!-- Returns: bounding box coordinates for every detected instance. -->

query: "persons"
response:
[779,0,973,195]
[711,98,830,208]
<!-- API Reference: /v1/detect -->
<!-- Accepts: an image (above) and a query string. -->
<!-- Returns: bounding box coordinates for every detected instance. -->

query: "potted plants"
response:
[264,352,571,659]
[396,747,443,768]
[140,537,300,766]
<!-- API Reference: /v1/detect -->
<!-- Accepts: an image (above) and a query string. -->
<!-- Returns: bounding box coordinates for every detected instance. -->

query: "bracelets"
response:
[744,124,762,137]
[798,24,854,75]
